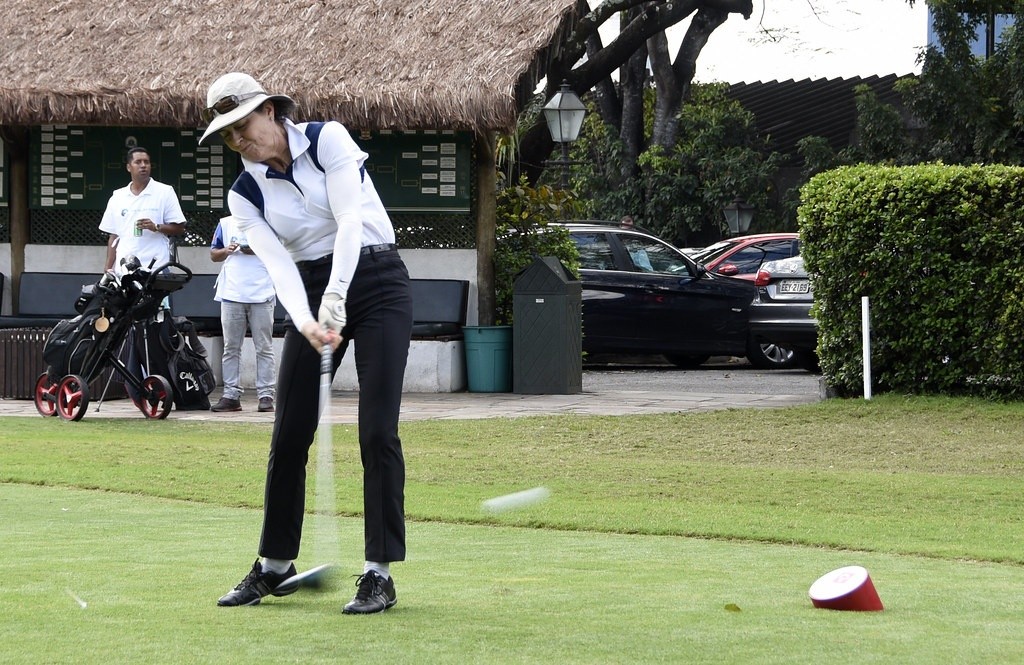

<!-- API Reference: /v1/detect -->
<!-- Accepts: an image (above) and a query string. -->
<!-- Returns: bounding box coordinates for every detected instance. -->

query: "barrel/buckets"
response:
[462,325,512,392]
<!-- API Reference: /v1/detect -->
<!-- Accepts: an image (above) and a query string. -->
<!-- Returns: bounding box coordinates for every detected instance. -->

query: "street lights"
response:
[540,77,589,192]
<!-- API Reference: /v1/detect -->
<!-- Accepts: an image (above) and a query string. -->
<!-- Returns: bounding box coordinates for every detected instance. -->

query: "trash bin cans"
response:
[511,255,583,394]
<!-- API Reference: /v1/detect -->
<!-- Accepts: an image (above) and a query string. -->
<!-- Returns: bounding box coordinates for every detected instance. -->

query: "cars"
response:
[690,232,804,280]
[748,241,880,373]
[676,247,707,259]
[406,217,754,371]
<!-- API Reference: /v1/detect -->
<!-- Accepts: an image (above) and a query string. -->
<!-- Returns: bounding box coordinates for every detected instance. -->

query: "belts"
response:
[302,242,398,267]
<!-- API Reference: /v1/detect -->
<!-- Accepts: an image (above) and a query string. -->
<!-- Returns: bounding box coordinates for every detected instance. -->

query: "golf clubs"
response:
[81,253,153,298]
[275,343,336,591]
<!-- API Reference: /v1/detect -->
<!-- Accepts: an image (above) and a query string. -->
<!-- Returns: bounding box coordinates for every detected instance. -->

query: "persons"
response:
[210,215,277,413]
[198,71,414,616]
[99,148,188,409]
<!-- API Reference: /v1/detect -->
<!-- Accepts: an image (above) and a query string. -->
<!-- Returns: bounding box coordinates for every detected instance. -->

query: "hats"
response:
[198,72,297,145]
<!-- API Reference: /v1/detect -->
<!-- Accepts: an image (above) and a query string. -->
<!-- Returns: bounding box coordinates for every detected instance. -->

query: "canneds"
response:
[134,220,142,236]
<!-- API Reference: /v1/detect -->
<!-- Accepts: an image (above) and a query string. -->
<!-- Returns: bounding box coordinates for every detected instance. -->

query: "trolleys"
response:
[32,253,193,421]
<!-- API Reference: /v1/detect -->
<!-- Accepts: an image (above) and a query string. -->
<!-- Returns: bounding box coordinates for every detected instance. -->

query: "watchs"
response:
[155,224,161,232]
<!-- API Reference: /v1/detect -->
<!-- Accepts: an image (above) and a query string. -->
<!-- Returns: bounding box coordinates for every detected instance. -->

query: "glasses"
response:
[199,92,266,125]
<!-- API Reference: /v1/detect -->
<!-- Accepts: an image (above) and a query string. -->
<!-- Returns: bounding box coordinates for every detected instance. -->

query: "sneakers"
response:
[258,396,274,412]
[211,397,242,412]
[217,558,299,606]
[341,570,398,614]
